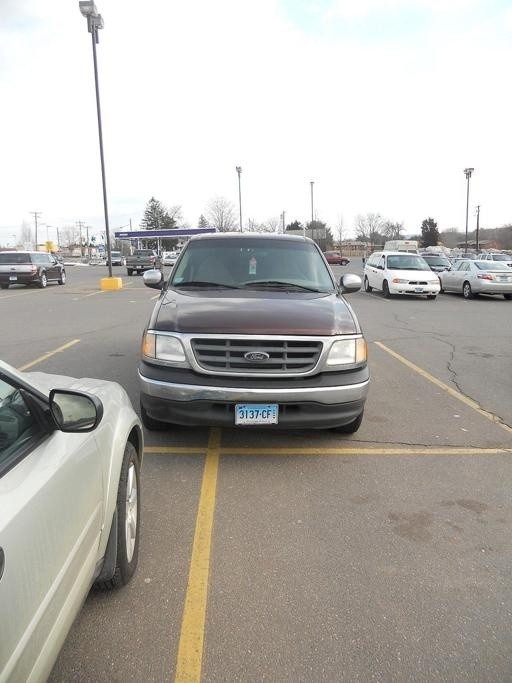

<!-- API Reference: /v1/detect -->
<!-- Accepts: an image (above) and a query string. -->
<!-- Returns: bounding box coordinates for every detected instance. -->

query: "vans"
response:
[364,251,440,299]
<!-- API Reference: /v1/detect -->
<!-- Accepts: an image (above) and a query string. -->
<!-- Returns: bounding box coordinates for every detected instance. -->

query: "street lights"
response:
[464,167,474,251]
[236,166,243,231]
[77,0,113,280]
[310,181,314,221]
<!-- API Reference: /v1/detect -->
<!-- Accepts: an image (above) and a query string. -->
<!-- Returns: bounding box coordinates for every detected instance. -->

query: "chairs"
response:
[195,255,233,285]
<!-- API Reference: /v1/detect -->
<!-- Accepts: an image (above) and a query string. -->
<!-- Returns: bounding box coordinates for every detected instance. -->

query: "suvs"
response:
[1,250,65,288]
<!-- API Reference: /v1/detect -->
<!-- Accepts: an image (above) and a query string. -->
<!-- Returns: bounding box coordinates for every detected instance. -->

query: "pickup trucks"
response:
[126,248,163,275]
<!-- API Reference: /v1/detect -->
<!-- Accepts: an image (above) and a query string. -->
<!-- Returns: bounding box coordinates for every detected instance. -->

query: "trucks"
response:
[385,239,417,252]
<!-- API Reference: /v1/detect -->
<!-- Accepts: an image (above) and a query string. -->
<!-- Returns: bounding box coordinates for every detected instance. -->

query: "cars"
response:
[323,249,350,265]
[106,249,124,264]
[437,259,512,299]
[421,251,451,271]
[163,253,178,265]
[137,232,370,432]
[453,249,512,266]
[1,359,144,683]
